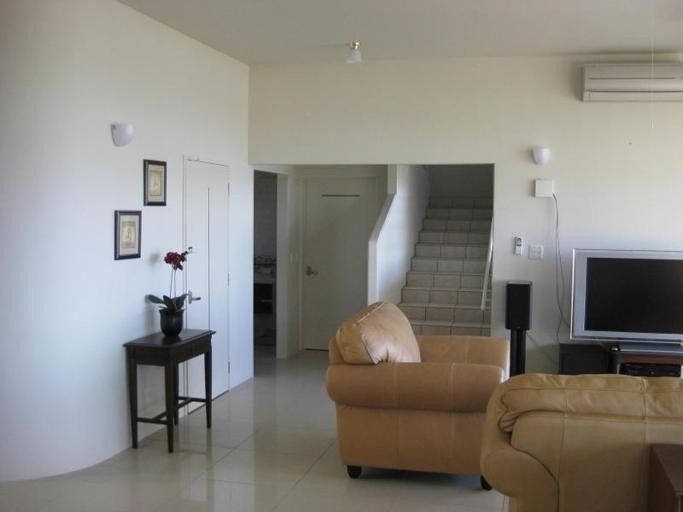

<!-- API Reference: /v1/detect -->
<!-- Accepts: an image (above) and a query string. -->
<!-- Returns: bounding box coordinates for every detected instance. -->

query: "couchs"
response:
[479,372,683,512]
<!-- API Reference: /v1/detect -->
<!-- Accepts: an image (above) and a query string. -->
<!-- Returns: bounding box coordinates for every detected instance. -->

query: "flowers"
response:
[149,247,200,311]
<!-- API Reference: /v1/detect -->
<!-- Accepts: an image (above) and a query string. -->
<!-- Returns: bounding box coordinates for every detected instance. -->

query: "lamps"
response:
[346,41,361,64]
[532,148,551,164]
[112,122,136,146]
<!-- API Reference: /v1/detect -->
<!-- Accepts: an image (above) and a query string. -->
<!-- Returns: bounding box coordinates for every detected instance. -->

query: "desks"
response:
[647,442,683,511]
[123,328,216,452]
[605,343,683,375]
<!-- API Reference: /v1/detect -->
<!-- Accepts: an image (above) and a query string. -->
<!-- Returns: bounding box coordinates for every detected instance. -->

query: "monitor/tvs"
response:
[570,248,683,345]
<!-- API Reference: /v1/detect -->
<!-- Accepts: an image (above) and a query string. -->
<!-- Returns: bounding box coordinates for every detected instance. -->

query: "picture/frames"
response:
[144,159,167,205]
[114,210,140,260]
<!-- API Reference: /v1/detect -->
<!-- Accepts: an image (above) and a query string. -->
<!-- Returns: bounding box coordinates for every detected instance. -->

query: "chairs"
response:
[325,334,509,490]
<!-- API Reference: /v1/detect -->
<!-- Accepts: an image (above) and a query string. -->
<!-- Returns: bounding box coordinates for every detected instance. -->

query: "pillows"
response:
[335,300,421,364]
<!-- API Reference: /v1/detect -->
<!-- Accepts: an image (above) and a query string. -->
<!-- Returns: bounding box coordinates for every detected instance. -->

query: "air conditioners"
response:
[582,60,683,102]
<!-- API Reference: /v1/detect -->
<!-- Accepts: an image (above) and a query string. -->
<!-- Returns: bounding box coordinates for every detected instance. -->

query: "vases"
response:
[159,309,184,337]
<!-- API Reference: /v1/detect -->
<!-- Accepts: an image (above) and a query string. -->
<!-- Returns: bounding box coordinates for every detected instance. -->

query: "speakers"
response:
[505,281,532,331]
